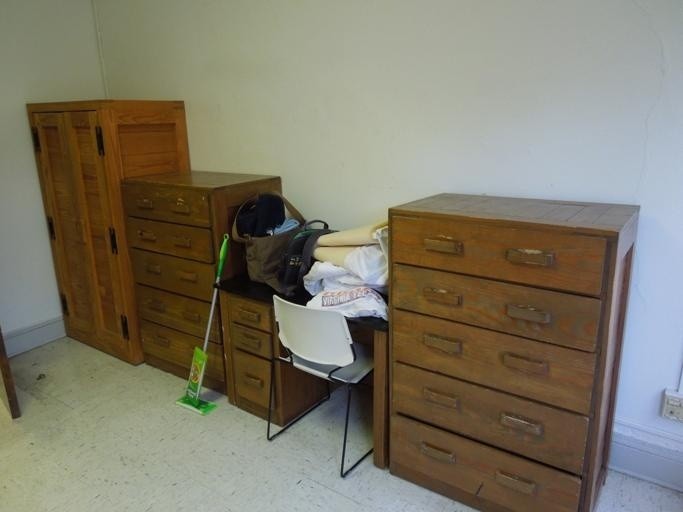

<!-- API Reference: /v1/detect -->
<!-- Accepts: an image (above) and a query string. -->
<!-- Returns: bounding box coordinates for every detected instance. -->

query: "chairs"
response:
[267,294,377,477]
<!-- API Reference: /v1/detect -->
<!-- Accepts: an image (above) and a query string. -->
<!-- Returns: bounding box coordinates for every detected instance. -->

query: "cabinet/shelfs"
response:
[120,170,279,400]
[386,192,643,512]
[25,99,191,367]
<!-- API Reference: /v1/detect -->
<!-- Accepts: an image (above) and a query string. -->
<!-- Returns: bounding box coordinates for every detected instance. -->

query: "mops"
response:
[174,234,231,417]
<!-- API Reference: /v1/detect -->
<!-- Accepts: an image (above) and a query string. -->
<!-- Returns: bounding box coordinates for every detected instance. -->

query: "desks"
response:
[213,269,388,469]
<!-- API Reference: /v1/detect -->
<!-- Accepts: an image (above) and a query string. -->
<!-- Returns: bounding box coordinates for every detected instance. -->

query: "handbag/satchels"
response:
[232,190,340,295]
